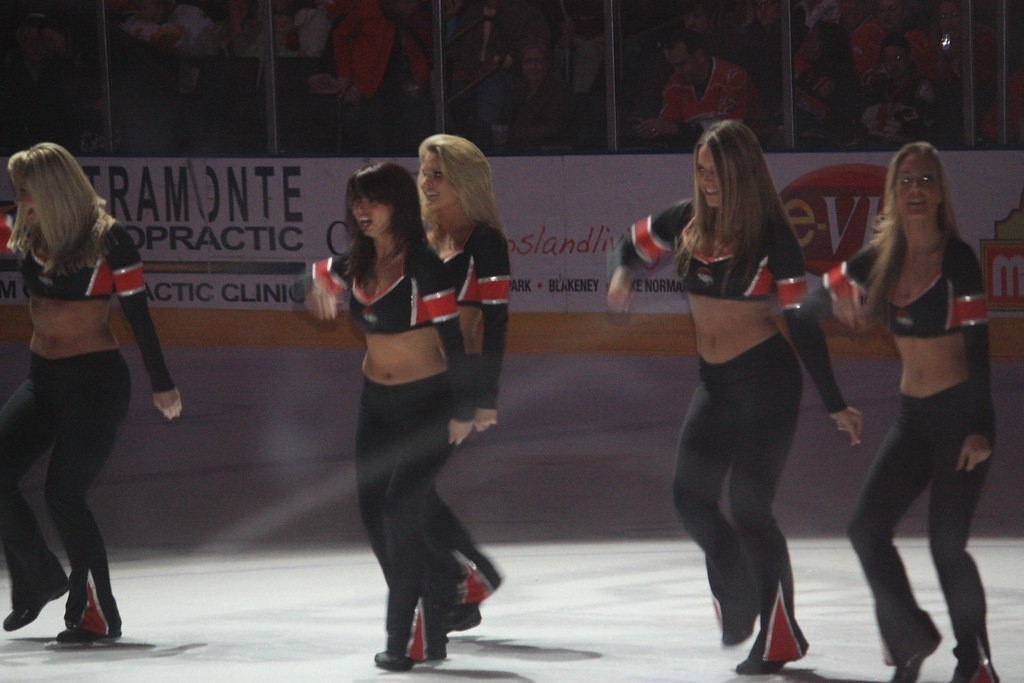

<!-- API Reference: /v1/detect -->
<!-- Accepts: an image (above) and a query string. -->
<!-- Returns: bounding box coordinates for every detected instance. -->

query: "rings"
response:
[651,127,656,133]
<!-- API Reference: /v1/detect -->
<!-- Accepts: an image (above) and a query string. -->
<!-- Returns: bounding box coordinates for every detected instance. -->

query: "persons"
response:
[307,160,476,672]
[798,142,1000,683]
[603,120,866,673]
[415,133,510,626]
[0,140,183,643]
[0,1,1024,152]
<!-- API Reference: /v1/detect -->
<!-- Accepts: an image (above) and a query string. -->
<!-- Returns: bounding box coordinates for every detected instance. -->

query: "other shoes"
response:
[374,647,449,670]
[58,629,122,643]
[722,619,755,645]
[4,576,68,631]
[440,603,481,634]
[736,658,786,676]
[889,624,942,683]
